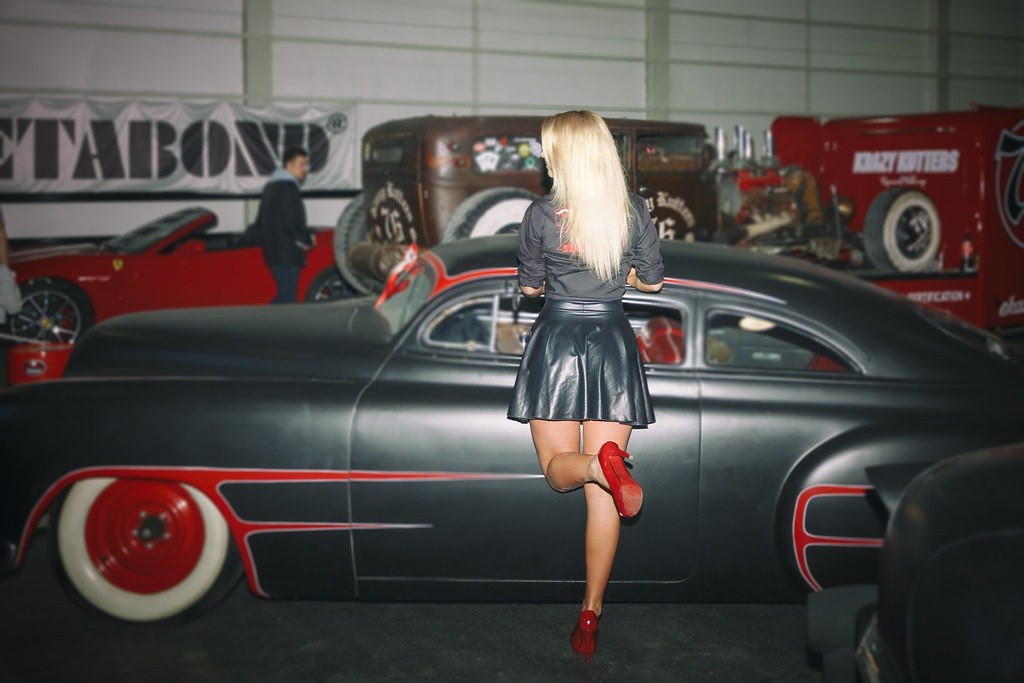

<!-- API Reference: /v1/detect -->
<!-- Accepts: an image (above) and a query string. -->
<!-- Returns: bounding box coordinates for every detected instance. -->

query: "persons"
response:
[506,110,665,665]
[256,146,314,305]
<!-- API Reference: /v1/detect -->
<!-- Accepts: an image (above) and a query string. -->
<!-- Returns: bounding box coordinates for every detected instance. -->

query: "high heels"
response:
[598,442,643,518]
[572,610,599,664]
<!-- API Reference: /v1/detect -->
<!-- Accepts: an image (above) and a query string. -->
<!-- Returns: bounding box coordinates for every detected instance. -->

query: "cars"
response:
[847,445,1024,683]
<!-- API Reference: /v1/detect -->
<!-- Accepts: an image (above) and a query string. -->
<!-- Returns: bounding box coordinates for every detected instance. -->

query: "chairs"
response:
[637,316,685,364]
[238,224,254,245]
[808,355,845,371]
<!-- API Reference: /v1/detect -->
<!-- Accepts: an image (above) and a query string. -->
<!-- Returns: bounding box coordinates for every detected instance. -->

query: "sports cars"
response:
[0,204,360,345]
[1,231,1024,645]
